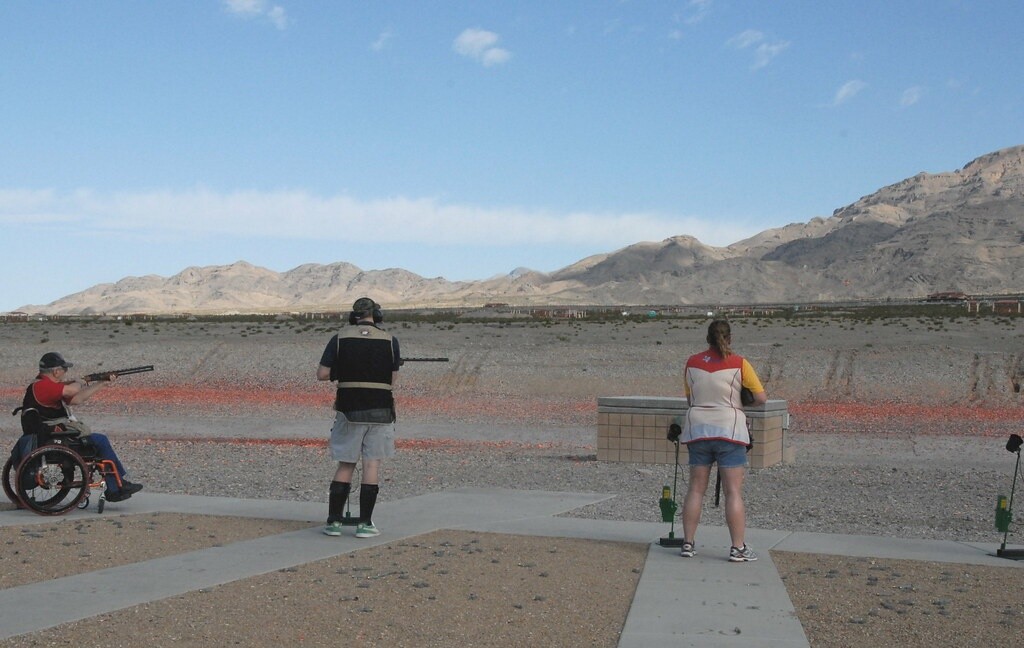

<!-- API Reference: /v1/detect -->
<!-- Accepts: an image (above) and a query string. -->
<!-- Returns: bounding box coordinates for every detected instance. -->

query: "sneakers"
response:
[679,539,697,556]
[729,542,759,563]
[323,520,343,537]
[355,520,381,538]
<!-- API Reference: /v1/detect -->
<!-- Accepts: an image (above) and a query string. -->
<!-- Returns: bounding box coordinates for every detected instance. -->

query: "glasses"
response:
[58,367,69,372]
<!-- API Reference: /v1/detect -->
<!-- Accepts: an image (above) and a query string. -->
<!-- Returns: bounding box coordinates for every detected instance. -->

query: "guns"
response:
[61,364,156,381]
[715,385,754,506]
[401,357,453,363]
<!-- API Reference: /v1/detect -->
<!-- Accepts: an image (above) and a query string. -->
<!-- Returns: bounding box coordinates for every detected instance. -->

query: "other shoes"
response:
[121,480,143,495]
[104,491,132,502]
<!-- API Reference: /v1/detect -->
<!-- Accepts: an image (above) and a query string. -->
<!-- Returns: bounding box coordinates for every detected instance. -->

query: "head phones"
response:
[349,309,383,325]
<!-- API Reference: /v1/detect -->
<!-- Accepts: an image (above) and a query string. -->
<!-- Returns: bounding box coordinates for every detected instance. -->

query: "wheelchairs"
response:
[2,406,131,517]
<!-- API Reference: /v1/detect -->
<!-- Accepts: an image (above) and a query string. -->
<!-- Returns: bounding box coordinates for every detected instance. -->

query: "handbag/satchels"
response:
[741,388,754,407]
[65,419,92,439]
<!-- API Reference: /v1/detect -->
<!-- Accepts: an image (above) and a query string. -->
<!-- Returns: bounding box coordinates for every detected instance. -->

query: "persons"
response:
[316,298,400,538]
[679,319,767,561]
[19,352,143,498]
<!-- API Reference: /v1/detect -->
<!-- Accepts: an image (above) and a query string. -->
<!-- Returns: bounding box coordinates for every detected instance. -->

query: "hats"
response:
[354,298,380,319]
[39,353,73,371]
